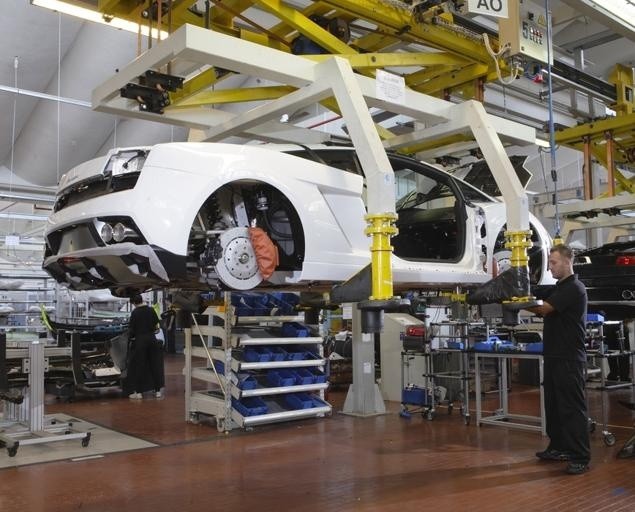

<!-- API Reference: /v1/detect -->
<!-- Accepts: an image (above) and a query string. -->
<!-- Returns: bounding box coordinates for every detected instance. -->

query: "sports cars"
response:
[569,239,635,313]
[41,142,555,304]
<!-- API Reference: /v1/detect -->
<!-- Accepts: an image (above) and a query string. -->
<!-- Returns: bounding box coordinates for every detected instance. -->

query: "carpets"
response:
[0,412,162,468]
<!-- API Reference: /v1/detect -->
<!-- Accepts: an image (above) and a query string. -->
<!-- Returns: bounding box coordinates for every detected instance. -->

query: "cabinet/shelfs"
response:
[182,290,333,433]
[399,350,454,419]
[428,321,490,427]
[582,319,635,447]
[474,349,548,437]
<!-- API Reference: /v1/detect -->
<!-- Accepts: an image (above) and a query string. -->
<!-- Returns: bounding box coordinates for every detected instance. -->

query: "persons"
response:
[522,243,590,474]
[166,310,176,353]
[126,295,163,399]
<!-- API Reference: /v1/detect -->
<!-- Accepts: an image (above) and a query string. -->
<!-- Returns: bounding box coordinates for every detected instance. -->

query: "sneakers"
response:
[128,391,143,399]
[536,447,569,460]
[567,460,588,474]
[153,390,162,398]
